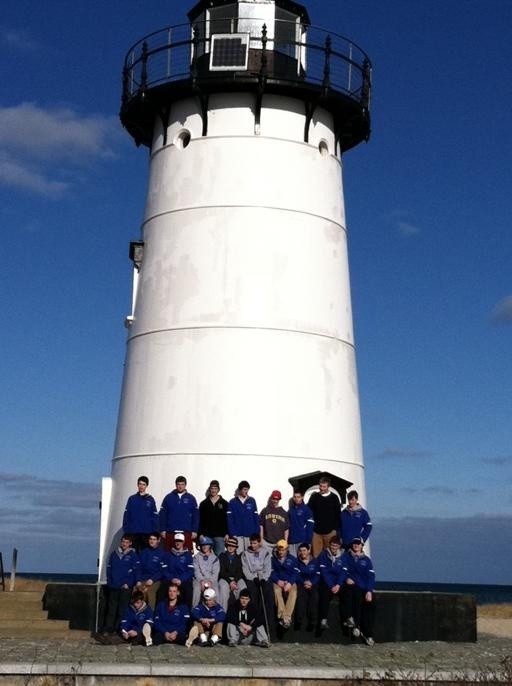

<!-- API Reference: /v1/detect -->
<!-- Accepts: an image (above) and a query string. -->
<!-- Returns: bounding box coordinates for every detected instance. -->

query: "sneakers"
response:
[143,623,153,646]
[261,640,271,648]
[344,618,354,628]
[185,626,199,647]
[353,628,361,637]
[361,633,374,646]
[94,633,112,644]
[228,641,237,646]
[320,624,329,629]
[280,616,291,628]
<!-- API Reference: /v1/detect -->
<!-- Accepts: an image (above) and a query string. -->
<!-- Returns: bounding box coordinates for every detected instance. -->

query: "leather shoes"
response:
[201,640,214,647]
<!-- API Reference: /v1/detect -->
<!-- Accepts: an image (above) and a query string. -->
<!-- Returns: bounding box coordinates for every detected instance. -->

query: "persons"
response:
[198,481,230,557]
[122,476,160,535]
[287,489,315,558]
[341,491,372,553]
[97,532,375,649]
[226,481,262,556]
[159,476,200,552]
[259,490,290,557]
[306,477,343,558]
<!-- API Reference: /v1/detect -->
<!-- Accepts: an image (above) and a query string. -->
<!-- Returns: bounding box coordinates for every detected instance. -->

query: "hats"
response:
[175,534,185,542]
[272,491,281,500]
[225,537,238,548]
[352,536,364,546]
[203,588,215,599]
[200,537,213,544]
[277,540,288,549]
